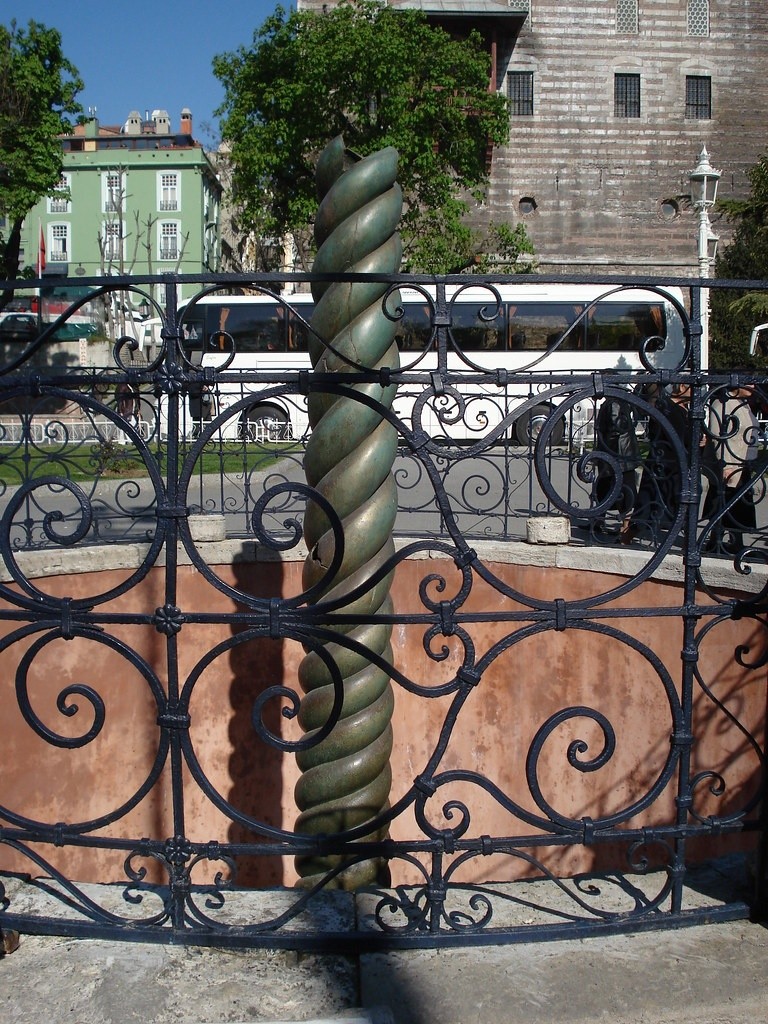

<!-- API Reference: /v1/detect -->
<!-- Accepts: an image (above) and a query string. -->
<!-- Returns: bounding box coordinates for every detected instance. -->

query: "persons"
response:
[91,363,211,434]
[582,364,759,556]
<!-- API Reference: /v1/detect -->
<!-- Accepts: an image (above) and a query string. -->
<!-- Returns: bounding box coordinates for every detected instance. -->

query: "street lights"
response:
[689,144,725,432]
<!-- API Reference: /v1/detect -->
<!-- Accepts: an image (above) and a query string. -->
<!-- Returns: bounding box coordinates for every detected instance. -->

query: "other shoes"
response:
[706,541,737,554]
[729,542,756,558]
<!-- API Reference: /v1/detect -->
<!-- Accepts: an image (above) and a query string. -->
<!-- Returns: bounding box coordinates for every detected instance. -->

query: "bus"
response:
[138,284,686,447]
